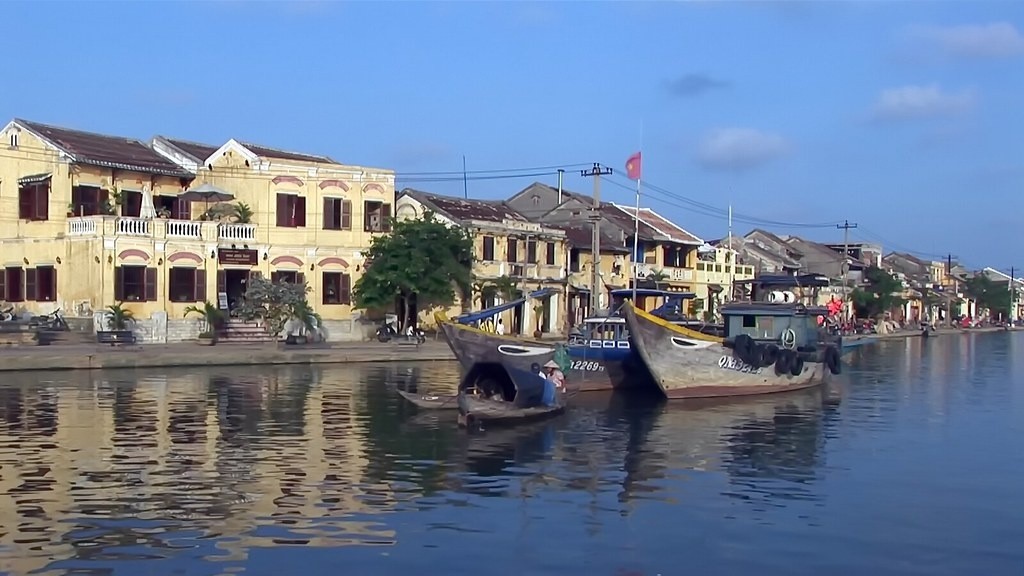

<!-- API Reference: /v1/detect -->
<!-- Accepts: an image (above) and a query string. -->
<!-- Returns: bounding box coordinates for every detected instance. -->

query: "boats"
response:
[624,270,844,401]
[840,334,878,355]
[396,388,463,410]
[454,357,581,425]
[433,283,726,391]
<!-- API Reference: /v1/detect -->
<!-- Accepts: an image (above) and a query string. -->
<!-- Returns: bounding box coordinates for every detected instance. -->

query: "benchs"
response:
[391,335,418,352]
[96,331,136,346]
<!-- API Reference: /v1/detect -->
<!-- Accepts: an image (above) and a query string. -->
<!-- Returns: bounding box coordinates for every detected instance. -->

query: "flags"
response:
[626,152,641,180]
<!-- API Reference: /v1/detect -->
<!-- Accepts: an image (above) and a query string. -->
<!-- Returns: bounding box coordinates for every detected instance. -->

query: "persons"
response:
[542,360,567,394]
[496,319,505,335]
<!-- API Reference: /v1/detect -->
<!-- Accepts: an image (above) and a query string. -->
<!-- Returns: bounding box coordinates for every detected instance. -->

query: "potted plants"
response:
[533,304,544,340]
[67,203,76,217]
[294,300,323,345]
[158,207,172,219]
[183,300,226,346]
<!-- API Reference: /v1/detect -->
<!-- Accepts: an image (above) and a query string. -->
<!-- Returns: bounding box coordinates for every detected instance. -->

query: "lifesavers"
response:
[735,334,758,365]
[755,342,778,367]
[826,345,842,374]
[791,351,804,377]
[776,348,790,374]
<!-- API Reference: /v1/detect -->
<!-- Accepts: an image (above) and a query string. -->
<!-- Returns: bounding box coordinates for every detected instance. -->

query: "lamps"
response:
[57,256,61,264]
[232,244,235,249]
[211,250,215,259]
[24,258,29,265]
[108,255,113,264]
[263,253,267,260]
[356,264,360,271]
[158,258,163,265]
[311,264,314,271]
[243,244,248,250]
[95,256,100,263]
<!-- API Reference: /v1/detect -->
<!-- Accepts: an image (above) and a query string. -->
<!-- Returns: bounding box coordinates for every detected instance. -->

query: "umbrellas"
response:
[139,185,158,219]
[177,183,236,220]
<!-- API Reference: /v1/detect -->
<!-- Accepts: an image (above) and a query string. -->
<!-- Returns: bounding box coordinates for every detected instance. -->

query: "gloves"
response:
[561,387,566,394]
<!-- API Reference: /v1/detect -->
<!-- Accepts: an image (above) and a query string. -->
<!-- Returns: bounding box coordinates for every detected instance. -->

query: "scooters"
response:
[407,319,427,345]
[374,318,402,343]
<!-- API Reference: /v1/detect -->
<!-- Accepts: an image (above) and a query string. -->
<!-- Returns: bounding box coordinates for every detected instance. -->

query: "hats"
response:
[544,360,559,368]
